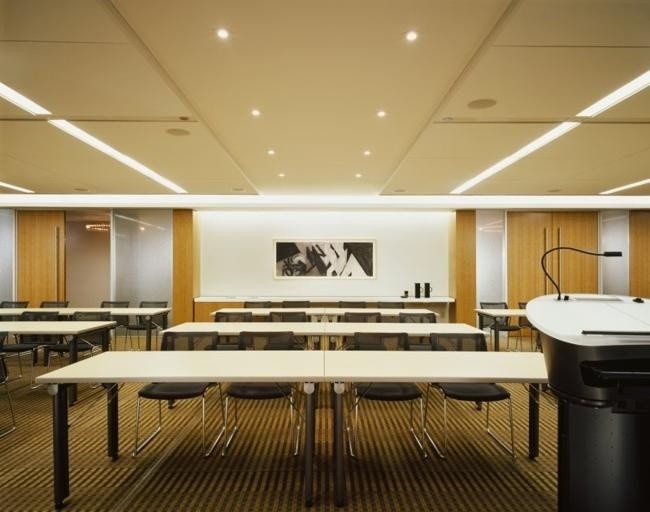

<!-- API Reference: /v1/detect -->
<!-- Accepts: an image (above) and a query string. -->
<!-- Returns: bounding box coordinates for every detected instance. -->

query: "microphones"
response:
[541,246,622,299]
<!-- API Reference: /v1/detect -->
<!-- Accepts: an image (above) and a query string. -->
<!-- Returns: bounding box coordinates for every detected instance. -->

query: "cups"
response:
[415,282,423,298]
[425,283,432,298]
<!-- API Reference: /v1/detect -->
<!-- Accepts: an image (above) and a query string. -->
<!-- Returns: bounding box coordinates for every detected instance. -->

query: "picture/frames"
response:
[272,237,377,283]
